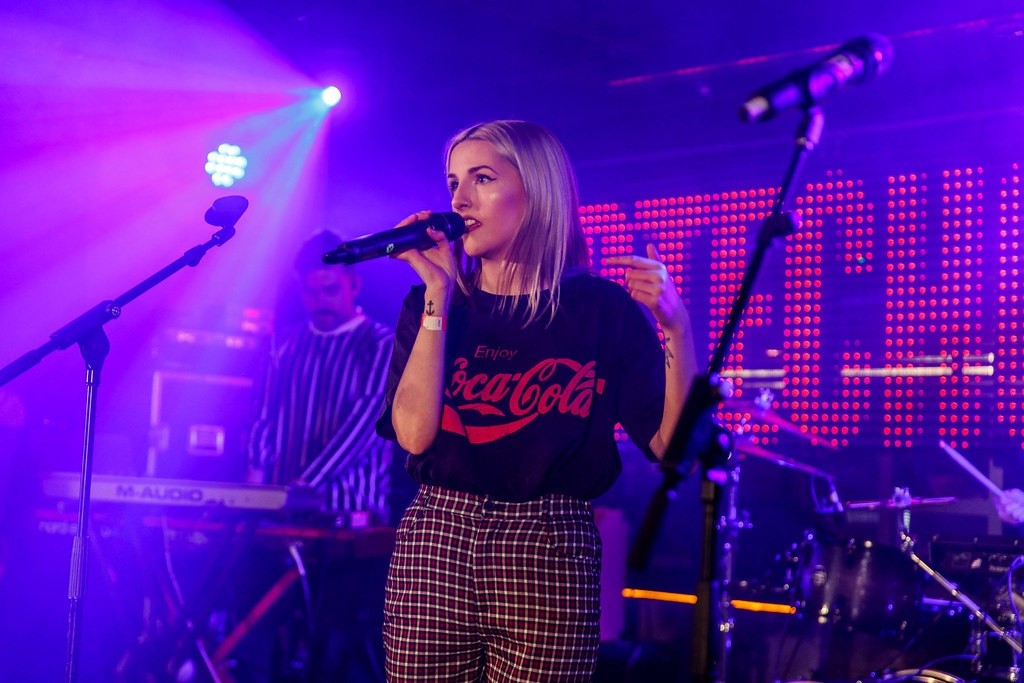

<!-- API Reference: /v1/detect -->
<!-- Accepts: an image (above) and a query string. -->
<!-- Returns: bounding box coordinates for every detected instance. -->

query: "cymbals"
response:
[717,428,837,486]
[720,395,839,452]
[811,493,959,518]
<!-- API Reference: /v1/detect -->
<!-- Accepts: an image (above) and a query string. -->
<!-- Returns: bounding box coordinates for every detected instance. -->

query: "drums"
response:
[970,606,1024,683]
[792,533,920,636]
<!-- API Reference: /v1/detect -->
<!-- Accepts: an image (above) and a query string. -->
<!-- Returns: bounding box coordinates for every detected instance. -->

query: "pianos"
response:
[31,468,343,517]
[22,502,401,564]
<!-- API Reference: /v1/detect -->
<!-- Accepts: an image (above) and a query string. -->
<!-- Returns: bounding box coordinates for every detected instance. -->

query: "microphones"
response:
[322,212,466,266]
[827,479,843,513]
[737,32,894,122]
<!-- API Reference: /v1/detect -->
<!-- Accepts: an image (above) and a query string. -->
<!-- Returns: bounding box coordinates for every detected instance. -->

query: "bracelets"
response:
[421,311,449,331]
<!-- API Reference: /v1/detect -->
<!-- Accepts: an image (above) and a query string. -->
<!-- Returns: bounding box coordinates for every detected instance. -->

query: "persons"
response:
[246,228,396,681]
[380,119,698,682]
